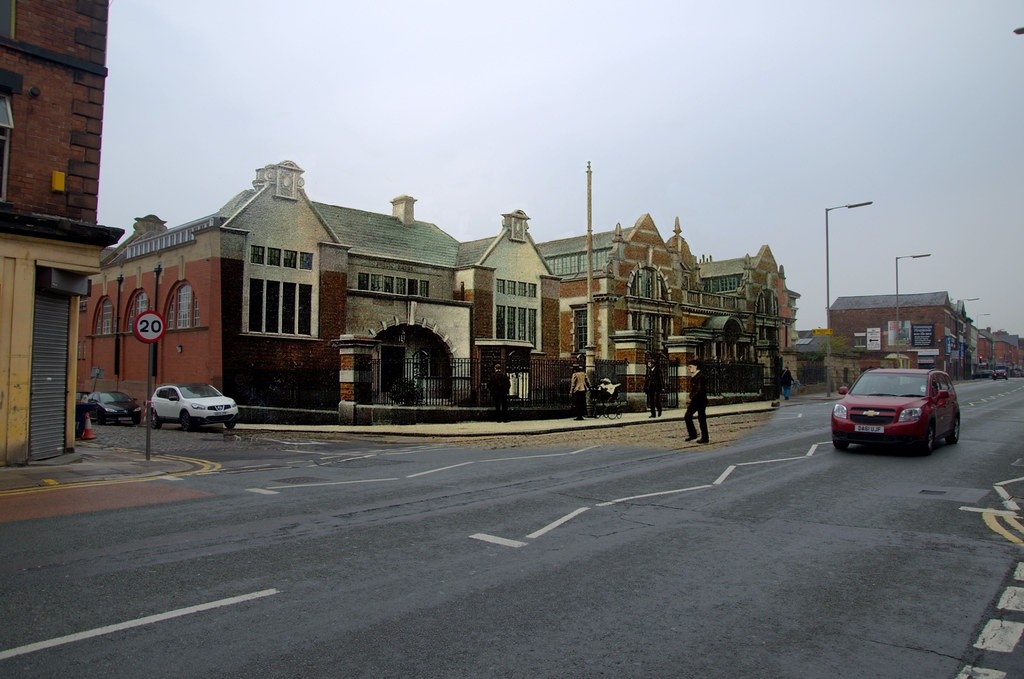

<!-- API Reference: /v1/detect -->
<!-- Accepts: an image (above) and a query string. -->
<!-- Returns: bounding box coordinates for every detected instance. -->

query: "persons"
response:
[684,359,709,444]
[643,360,665,418]
[490,364,511,423]
[781,365,795,400]
[569,364,594,420]
[598,378,615,394]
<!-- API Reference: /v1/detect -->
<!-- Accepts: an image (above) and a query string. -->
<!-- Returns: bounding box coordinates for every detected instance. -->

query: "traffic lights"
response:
[979,356,983,363]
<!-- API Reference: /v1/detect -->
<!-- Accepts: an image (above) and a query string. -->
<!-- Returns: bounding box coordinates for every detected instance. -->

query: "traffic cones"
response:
[80,412,97,440]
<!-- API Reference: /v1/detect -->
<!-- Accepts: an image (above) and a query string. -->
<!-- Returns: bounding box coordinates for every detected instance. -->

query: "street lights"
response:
[954,299,979,380]
[895,254,932,368]
[824,201,874,397]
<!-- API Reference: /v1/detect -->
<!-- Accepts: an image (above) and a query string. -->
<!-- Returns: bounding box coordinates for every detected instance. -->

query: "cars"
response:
[149,381,239,431]
[80,390,141,425]
[971,369,994,380]
[993,369,1008,381]
[831,367,961,456]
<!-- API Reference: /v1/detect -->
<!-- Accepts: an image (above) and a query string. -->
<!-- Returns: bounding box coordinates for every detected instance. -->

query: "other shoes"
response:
[685,435,698,441]
[697,439,708,443]
[649,414,656,418]
[658,410,662,417]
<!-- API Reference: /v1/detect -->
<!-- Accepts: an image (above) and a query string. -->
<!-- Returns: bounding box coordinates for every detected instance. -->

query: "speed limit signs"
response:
[134,310,167,344]
